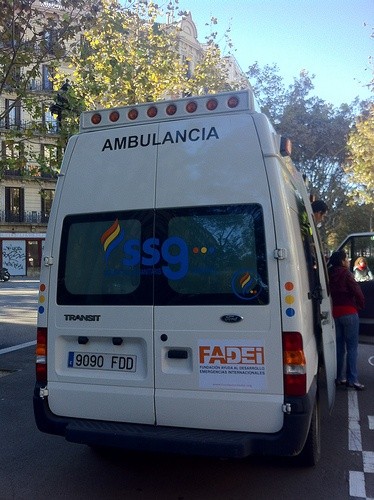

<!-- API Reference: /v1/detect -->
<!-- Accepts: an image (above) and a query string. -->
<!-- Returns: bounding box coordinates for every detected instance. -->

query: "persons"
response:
[352,256,374,283]
[327,251,366,390]
[310,198,325,226]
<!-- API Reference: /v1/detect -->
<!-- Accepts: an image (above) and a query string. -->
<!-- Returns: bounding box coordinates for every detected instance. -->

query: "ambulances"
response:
[32,88,374,466]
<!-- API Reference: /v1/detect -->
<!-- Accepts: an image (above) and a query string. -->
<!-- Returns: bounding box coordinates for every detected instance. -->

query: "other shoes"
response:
[347,382,365,390]
[337,379,348,386]
